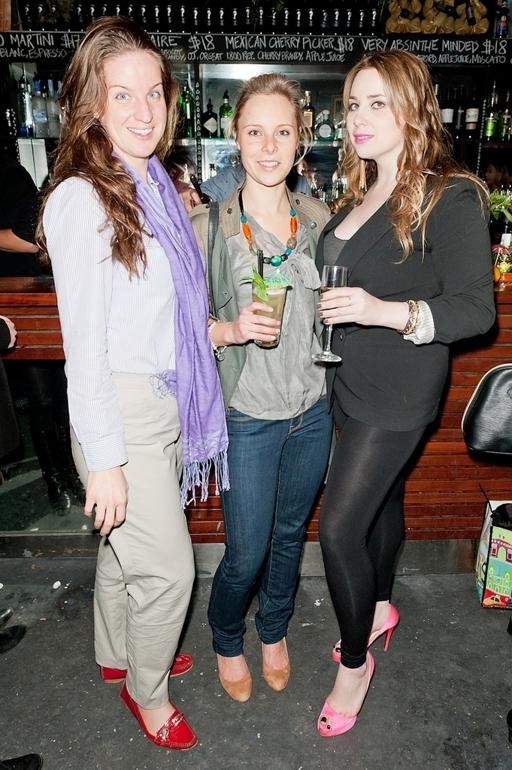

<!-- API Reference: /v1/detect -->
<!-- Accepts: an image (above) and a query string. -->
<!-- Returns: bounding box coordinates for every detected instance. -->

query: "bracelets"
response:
[403,296,418,341]
[207,320,228,361]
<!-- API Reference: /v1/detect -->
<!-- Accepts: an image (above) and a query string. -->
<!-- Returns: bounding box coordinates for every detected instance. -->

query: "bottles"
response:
[310,180,348,213]
[432,73,512,146]
[300,90,344,142]
[201,90,234,139]
[464,0,476,26]
[400,8,416,19]
[176,77,195,141]
[498,182,512,199]
[493,0,510,38]
[433,2,457,16]
[16,74,69,138]
[487,214,512,272]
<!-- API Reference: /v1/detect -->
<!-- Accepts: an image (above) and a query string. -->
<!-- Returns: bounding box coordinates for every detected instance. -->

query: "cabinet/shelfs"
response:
[4,55,512,198]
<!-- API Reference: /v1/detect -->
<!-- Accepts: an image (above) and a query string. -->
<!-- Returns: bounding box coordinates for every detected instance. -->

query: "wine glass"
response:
[312,264,348,363]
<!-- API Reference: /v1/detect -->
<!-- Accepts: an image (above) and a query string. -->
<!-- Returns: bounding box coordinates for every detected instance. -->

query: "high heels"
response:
[317,607,399,737]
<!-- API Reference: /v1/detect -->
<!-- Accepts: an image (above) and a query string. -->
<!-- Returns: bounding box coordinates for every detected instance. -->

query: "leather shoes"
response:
[100,654,193,684]
[121,680,198,750]
[261,636,290,691]
[219,673,252,703]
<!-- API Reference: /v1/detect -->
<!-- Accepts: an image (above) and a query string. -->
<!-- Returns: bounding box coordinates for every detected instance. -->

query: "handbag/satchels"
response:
[476,500,512,609]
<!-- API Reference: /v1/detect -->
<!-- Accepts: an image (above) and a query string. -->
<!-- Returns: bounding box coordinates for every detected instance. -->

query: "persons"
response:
[183,71,334,706]
[1,161,45,276]
[171,160,313,217]
[313,50,496,739]
[40,19,216,754]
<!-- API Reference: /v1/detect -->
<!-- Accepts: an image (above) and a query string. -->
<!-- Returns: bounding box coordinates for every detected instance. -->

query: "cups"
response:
[250,279,287,349]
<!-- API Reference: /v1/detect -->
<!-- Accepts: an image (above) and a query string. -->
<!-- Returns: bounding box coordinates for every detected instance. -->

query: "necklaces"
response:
[237,187,298,268]
